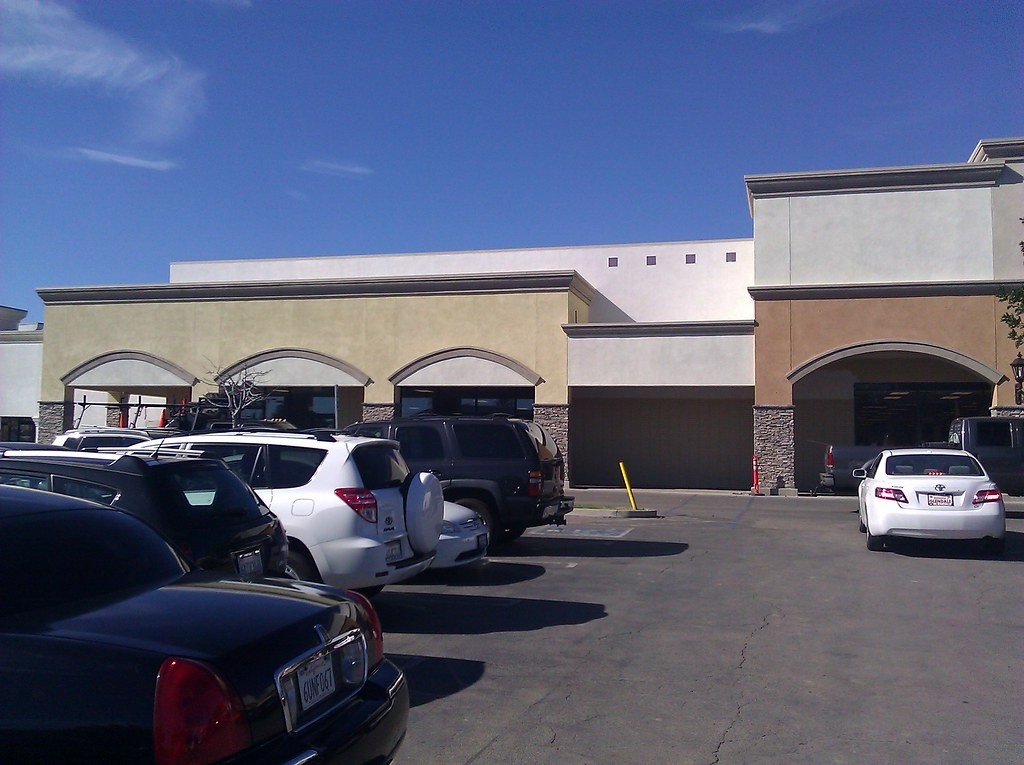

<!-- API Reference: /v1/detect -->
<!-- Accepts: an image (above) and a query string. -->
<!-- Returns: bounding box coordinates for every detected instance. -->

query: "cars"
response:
[0,484,411,765]
[853,447,1006,551]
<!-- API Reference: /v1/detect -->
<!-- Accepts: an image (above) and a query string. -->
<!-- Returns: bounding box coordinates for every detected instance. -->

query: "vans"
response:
[946,414,1024,499]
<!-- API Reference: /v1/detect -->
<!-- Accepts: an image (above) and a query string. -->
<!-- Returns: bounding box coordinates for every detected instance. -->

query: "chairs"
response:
[239,453,266,488]
[949,466,970,474]
[895,465,914,475]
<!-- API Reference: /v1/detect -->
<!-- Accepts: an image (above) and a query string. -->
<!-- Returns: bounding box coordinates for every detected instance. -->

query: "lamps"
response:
[1010,351,1024,404]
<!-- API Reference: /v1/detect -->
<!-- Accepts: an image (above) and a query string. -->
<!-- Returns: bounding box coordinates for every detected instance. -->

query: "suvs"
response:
[0,419,494,600]
[341,412,575,546]
